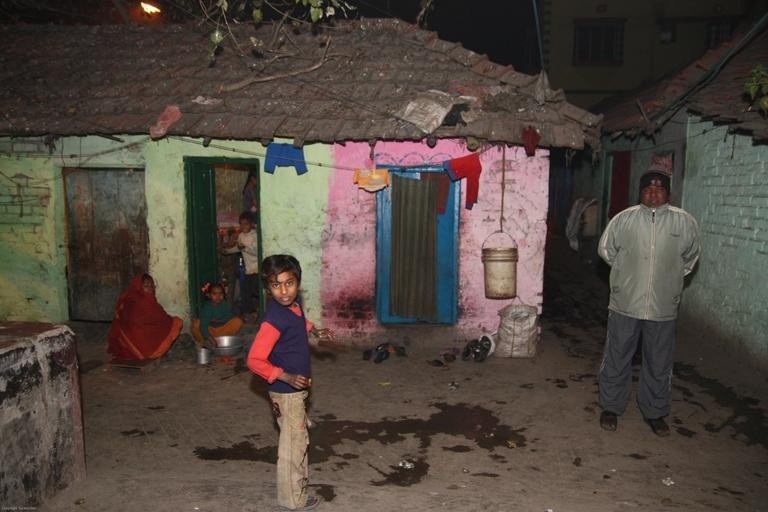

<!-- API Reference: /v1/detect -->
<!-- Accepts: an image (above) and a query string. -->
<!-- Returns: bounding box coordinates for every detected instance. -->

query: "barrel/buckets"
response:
[480,229,519,300]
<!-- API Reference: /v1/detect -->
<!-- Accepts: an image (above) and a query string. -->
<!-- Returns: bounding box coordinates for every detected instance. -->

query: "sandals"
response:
[303,495,318,509]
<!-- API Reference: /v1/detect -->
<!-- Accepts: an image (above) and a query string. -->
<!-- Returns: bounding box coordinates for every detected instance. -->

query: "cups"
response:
[197,348,209,365]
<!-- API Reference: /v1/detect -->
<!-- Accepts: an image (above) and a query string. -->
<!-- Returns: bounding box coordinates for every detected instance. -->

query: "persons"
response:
[219,212,260,323]
[594,171,702,435]
[247,255,337,511]
[106,272,183,362]
[190,282,244,349]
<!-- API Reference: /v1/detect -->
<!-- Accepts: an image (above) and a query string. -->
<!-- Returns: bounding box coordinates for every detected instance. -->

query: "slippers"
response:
[426,360,443,366]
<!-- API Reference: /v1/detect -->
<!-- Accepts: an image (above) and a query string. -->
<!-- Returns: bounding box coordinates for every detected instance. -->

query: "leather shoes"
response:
[364,343,405,363]
[649,418,669,436]
[464,336,490,361]
[600,410,617,430]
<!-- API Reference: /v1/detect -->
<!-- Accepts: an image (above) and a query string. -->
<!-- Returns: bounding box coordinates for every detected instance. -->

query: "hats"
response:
[639,160,669,189]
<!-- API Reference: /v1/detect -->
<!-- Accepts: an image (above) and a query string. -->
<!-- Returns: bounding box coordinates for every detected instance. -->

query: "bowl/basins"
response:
[211,335,247,355]
